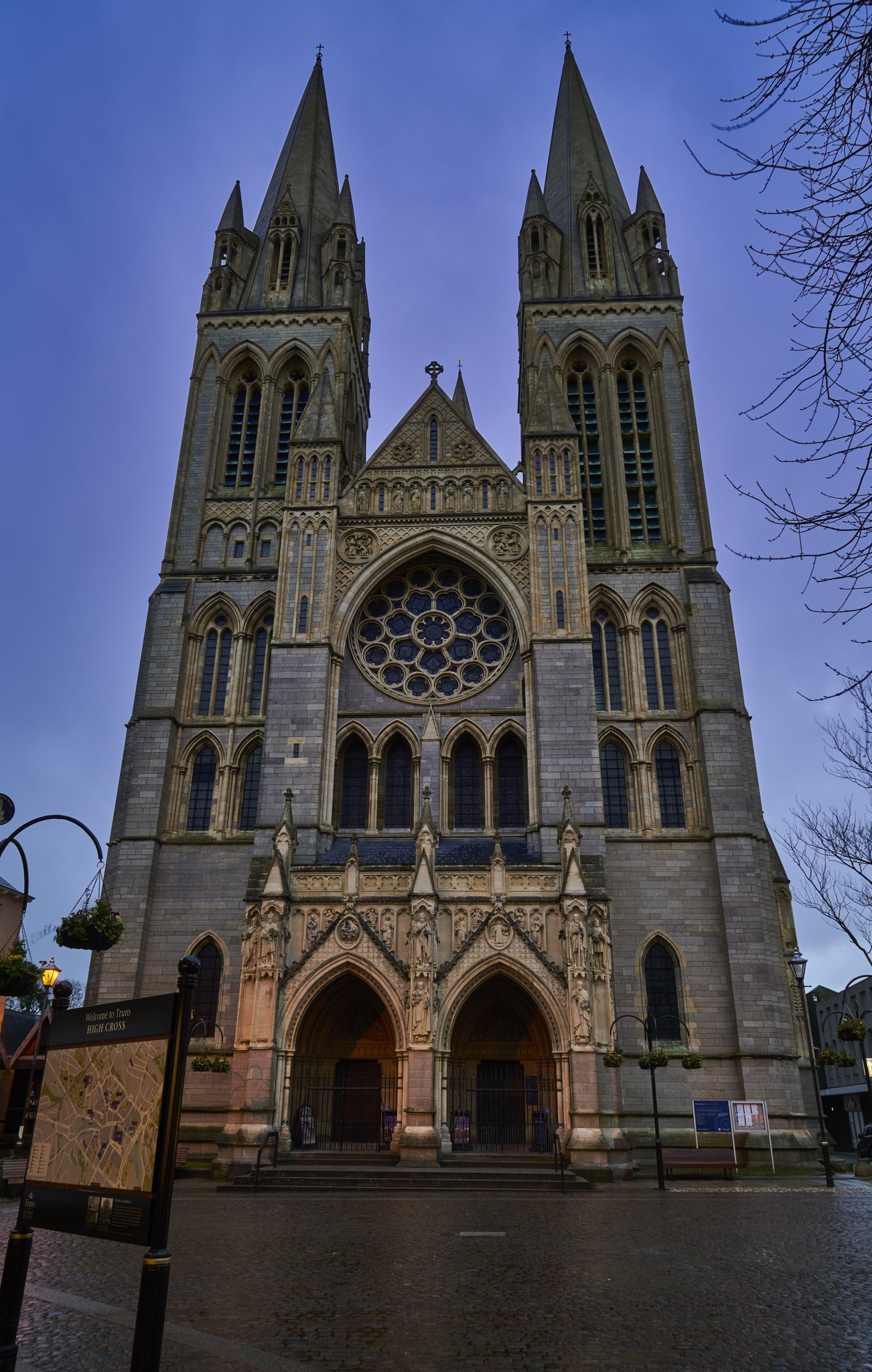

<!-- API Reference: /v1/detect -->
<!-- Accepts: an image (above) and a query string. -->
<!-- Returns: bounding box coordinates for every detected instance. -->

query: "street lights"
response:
[11,955,62,1159]
[787,946,834,1188]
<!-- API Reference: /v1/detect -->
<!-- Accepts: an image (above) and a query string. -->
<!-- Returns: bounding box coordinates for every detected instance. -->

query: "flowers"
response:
[190,1053,231,1074]
[816,1016,868,1067]
[53,896,125,946]
[682,1051,703,1069]
[602,1051,624,1067]
[0,936,45,992]
[638,1048,669,1067]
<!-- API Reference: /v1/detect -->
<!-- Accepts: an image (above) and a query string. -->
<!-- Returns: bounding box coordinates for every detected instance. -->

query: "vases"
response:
[605,1060,620,1068]
[0,972,37,996]
[194,1063,209,1072]
[212,1066,225,1073]
[822,1056,835,1065]
[682,1060,696,1069]
[649,1059,668,1067]
[841,1029,864,1041]
[637,1062,656,1070]
[840,1059,855,1067]
[62,922,120,951]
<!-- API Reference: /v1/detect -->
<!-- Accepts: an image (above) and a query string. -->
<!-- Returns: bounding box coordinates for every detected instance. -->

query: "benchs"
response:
[2,1157,28,1198]
[173,1146,190,1179]
[662,1146,739,1181]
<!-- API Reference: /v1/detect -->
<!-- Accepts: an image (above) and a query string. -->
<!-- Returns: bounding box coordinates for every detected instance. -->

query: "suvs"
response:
[857,1123,872,1158]
